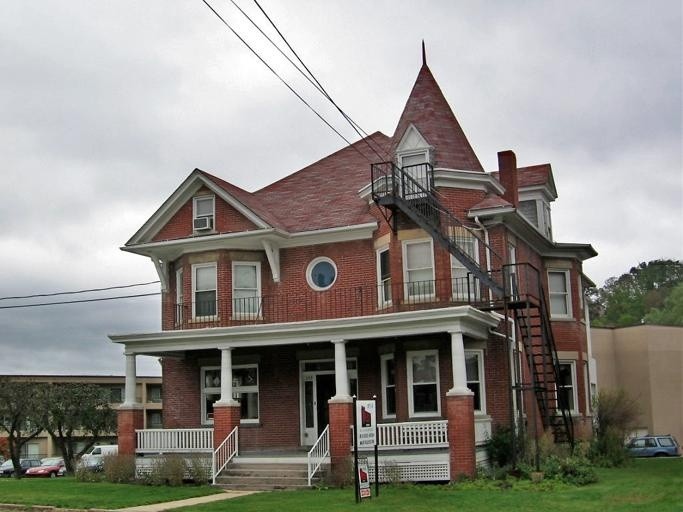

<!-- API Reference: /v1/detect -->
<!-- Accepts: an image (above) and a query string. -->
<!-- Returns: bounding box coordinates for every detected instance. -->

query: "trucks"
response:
[84,444,117,457]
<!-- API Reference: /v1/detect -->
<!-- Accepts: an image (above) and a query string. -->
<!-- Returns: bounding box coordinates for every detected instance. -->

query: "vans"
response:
[624,432,679,458]
[0,457,42,477]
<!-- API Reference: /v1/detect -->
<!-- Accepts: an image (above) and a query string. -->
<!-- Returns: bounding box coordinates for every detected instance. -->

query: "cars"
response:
[25,455,66,478]
[77,458,104,472]
